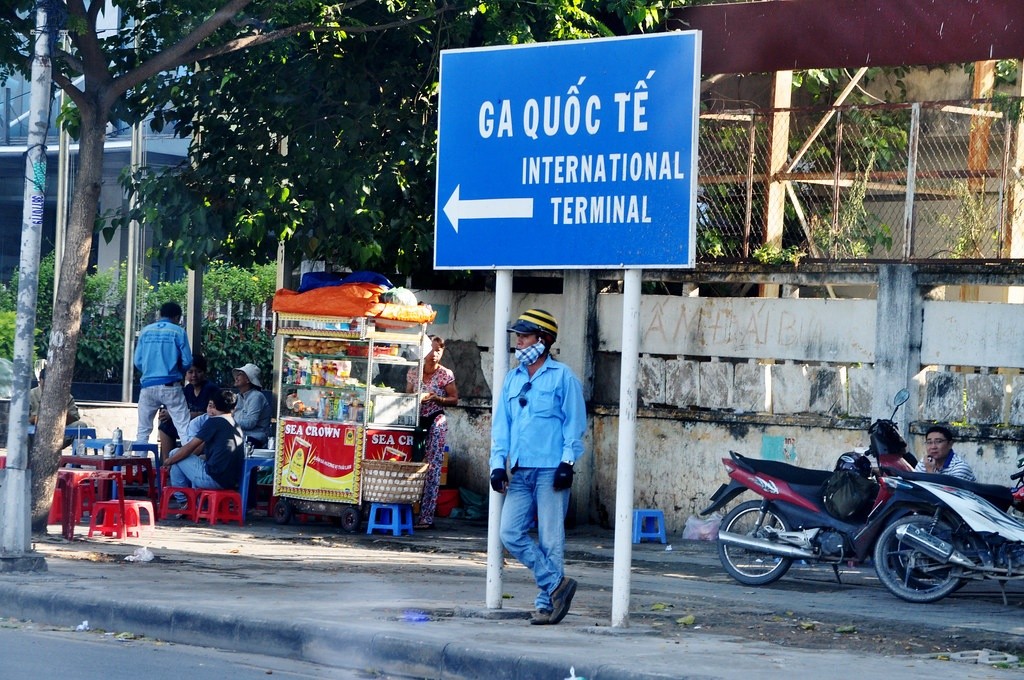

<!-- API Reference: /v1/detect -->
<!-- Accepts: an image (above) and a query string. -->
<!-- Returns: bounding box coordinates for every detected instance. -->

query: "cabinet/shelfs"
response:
[282,337,424,428]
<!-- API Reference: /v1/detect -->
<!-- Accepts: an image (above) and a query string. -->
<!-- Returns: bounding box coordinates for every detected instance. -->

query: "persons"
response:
[158,354,220,460]
[134,302,191,474]
[28,369,87,468]
[232,363,271,448]
[914,426,976,482]
[488,309,587,625]
[162,390,245,519]
[405,335,458,529]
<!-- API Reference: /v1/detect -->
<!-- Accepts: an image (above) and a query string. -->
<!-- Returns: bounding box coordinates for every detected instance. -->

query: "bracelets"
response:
[441,398,445,404]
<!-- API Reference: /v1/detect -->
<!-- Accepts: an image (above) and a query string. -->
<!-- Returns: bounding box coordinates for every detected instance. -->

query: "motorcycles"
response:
[699,388,919,587]
[873,458,1024,605]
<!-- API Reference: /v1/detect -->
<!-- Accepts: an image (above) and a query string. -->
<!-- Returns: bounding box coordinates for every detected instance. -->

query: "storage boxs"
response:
[422,446,449,486]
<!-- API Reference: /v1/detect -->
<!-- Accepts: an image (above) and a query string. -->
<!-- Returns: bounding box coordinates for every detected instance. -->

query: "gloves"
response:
[553,462,573,491]
[490,468,508,494]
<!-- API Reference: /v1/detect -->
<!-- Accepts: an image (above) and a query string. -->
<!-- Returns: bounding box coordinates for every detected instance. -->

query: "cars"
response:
[0,357,18,447]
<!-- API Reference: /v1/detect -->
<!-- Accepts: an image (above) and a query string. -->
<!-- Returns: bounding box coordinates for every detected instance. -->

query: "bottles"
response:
[283,361,365,421]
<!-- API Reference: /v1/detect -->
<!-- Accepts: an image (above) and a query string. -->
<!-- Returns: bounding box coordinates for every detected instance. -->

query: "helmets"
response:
[507,309,558,342]
[834,451,872,478]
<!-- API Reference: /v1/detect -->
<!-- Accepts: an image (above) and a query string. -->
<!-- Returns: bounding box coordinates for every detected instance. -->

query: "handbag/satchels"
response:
[413,411,445,461]
[682,511,723,541]
[823,469,881,525]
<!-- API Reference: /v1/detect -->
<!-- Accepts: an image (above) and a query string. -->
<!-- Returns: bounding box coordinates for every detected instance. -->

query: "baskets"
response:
[362,459,429,504]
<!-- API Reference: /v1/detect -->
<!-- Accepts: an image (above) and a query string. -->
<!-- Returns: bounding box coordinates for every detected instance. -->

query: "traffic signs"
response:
[432,30,703,271]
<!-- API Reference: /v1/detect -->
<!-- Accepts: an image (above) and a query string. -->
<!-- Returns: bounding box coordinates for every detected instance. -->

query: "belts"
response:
[164,382,174,386]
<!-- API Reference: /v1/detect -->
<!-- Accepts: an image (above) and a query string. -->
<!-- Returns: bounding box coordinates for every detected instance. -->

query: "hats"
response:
[232,362,262,388]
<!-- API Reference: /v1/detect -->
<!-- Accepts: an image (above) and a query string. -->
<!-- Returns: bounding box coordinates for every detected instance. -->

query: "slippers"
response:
[415,521,436,530]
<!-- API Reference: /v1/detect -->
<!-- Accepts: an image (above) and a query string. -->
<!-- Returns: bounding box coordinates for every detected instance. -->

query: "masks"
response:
[514,338,545,367]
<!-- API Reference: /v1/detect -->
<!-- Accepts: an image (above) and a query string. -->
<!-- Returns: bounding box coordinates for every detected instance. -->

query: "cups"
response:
[123,442,132,458]
[75,446,85,456]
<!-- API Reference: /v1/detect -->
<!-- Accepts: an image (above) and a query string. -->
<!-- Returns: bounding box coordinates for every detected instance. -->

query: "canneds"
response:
[287,439,311,486]
[382,448,406,462]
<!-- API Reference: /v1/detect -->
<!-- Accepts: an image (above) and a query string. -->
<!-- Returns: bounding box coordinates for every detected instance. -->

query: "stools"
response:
[47,455,241,539]
[251,486,276,517]
[366,503,415,537]
[631,507,668,545]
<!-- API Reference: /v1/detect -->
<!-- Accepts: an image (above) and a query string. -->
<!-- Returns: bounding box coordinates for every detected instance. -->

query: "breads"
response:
[284,338,351,354]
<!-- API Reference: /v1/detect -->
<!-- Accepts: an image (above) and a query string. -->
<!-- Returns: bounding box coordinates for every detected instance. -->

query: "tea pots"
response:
[104,443,117,457]
[112,427,122,442]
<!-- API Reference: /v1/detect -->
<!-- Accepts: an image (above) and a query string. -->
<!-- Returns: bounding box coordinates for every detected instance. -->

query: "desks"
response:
[70,439,162,500]
[26,426,99,467]
[61,455,157,524]
[240,456,275,522]
[55,469,124,542]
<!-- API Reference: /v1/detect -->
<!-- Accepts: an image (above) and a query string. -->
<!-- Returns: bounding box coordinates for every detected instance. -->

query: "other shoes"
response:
[175,503,188,519]
[247,509,263,521]
[549,577,577,624]
[531,608,552,624]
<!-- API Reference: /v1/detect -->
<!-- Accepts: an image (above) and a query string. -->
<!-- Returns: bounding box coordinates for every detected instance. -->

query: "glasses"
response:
[519,382,532,408]
[924,438,948,445]
